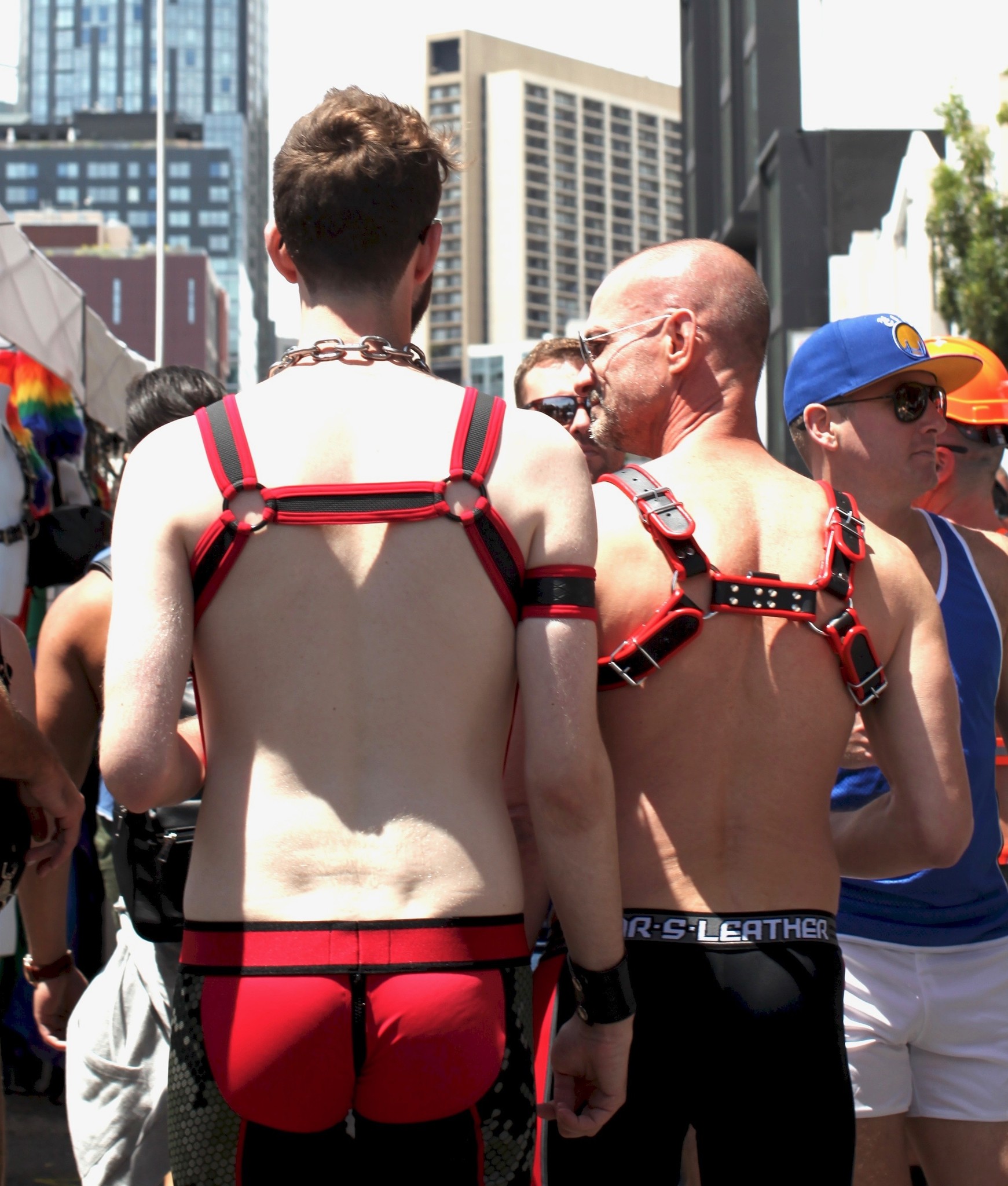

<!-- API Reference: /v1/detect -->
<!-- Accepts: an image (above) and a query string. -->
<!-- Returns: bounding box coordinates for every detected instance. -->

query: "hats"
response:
[783,313,983,427]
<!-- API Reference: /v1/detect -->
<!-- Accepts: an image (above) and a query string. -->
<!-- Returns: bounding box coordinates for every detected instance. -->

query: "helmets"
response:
[923,335,1008,447]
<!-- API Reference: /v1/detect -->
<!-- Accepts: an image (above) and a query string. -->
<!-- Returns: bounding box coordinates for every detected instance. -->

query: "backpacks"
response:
[91,547,204,944]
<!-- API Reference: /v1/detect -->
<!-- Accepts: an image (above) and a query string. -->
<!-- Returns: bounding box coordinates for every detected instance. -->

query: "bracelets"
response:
[567,948,637,1026]
[23,949,75,987]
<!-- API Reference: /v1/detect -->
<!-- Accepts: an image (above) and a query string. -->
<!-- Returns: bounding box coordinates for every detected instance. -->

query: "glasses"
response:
[577,315,701,364]
[796,381,947,431]
[521,395,593,427]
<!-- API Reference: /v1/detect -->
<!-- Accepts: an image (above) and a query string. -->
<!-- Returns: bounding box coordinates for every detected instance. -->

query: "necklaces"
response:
[268,335,431,379]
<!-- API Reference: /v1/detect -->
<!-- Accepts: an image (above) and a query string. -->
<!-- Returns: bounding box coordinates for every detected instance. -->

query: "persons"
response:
[99,84,636,1186]
[503,238,1008,1186]
[0,365,234,1186]
